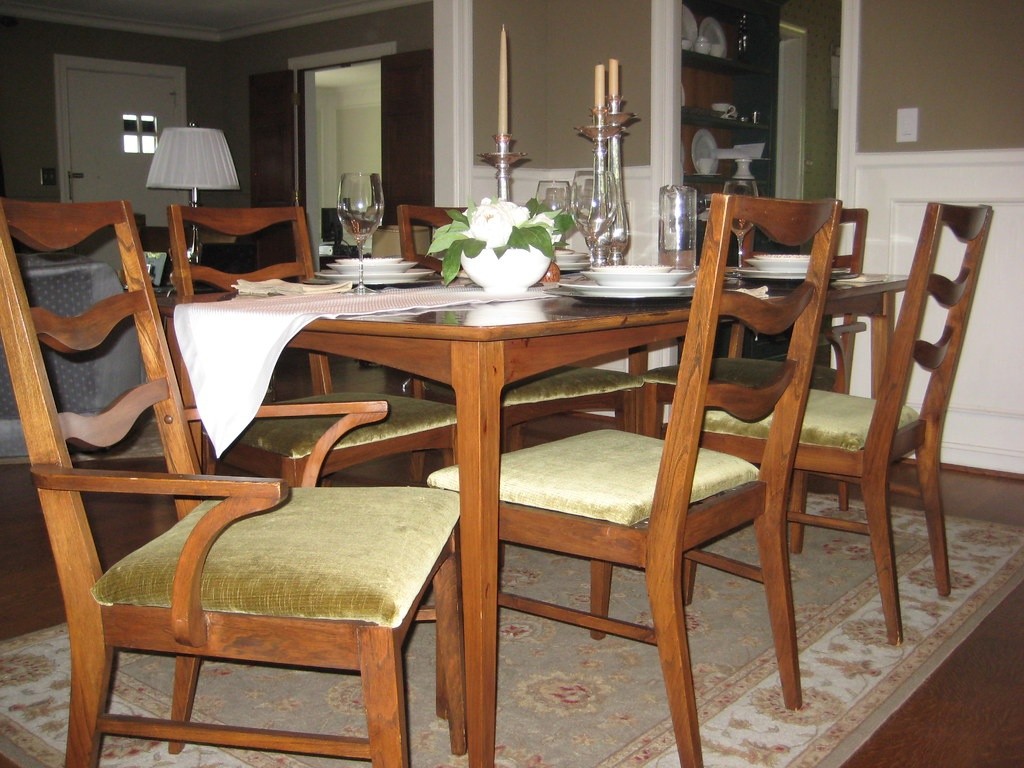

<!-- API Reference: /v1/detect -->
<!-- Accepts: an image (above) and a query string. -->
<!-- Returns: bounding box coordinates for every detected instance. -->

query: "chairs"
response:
[681,202,995,645]
[0,199,466,768]
[644,207,867,553]
[168,205,457,624]
[429,192,844,768]
[397,206,648,570]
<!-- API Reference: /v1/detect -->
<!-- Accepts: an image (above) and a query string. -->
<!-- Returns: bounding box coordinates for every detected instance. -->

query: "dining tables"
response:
[163,256,910,768]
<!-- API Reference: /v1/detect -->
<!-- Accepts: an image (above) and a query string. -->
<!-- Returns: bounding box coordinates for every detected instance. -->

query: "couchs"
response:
[0,252,141,458]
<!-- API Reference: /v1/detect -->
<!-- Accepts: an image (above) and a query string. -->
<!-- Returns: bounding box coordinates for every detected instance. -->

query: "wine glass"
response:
[581,178,628,266]
[722,180,759,278]
[336,172,385,296]
[571,170,618,270]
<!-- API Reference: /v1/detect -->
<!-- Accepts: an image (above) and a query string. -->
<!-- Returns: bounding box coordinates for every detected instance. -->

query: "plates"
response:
[580,271,694,286]
[589,264,674,273]
[738,254,849,280]
[698,17,727,59]
[681,4,698,52]
[543,287,695,302]
[556,280,696,296]
[691,128,718,175]
[314,258,436,284]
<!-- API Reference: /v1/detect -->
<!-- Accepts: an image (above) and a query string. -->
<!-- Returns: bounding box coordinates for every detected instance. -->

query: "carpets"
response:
[0,488,1024,768]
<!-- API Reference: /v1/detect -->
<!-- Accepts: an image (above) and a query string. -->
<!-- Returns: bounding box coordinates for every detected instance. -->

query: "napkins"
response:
[231,278,353,296]
[724,286,771,299]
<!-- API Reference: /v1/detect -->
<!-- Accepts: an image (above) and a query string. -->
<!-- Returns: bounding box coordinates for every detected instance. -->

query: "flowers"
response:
[423,197,576,287]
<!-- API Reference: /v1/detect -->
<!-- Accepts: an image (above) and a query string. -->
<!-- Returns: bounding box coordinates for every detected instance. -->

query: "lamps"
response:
[146,123,240,263]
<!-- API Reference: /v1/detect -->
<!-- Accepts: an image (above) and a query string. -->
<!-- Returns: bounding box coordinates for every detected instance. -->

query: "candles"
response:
[608,57,619,102]
[497,25,507,135]
[594,61,607,113]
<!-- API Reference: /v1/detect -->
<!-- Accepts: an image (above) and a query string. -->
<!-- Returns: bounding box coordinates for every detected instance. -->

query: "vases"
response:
[460,246,556,296]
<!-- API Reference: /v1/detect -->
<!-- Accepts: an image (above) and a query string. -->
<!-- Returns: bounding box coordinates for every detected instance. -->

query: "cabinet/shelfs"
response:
[681,0,780,266]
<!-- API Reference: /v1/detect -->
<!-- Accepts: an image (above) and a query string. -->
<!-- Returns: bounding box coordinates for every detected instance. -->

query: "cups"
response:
[535,180,571,215]
[711,102,736,119]
[681,39,692,51]
[656,184,697,270]
[710,43,724,58]
[695,42,710,55]
